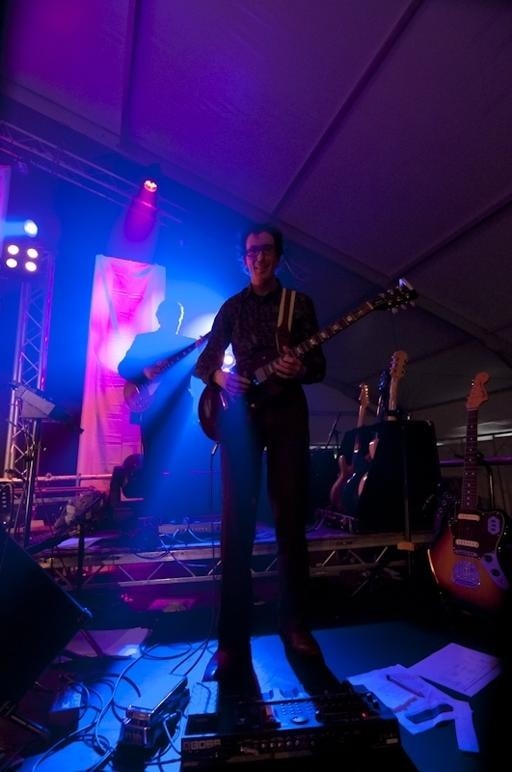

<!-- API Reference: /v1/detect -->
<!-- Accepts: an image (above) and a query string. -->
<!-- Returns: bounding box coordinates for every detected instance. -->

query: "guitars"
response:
[198,277,418,443]
[330,350,446,531]
[425,370,511,610]
[123,331,211,411]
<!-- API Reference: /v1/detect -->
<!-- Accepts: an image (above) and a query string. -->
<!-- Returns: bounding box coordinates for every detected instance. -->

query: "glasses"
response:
[246,244,274,257]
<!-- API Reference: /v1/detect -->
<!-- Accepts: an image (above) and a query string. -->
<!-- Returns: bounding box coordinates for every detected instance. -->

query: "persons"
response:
[117,300,208,525]
[194,224,323,644]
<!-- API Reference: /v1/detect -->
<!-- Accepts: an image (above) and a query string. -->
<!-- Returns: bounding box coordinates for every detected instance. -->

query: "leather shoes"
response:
[282,618,320,656]
[203,641,251,682]
